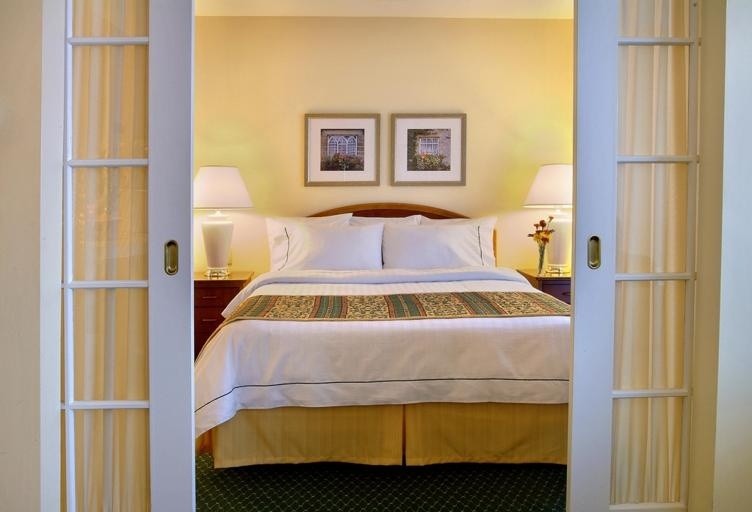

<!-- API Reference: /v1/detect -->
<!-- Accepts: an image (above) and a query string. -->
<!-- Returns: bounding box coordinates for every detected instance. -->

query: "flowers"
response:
[526,216,557,272]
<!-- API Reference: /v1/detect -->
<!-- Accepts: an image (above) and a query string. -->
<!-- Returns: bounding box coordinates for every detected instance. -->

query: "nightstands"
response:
[193,271,253,357]
[516,268,571,304]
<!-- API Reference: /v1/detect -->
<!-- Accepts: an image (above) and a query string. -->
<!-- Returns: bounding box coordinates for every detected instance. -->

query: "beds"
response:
[194,202,573,472]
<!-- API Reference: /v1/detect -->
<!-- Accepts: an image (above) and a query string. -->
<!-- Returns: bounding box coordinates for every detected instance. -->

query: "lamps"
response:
[523,162,574,274]
[193,166,254,277]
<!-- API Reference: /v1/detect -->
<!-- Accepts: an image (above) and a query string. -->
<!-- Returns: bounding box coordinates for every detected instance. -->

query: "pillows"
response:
[381,216,489,271]
[260,210,354,272]
[421,214,498,269]
[349,212,422,265]
[279,221,385,270]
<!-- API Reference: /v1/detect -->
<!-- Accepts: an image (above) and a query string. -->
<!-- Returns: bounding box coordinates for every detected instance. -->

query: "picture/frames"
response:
[303,111,383,189]
[391,111,468,188]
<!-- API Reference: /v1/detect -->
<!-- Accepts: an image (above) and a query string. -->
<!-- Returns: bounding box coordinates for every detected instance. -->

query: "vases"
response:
[534,242,545,276]
